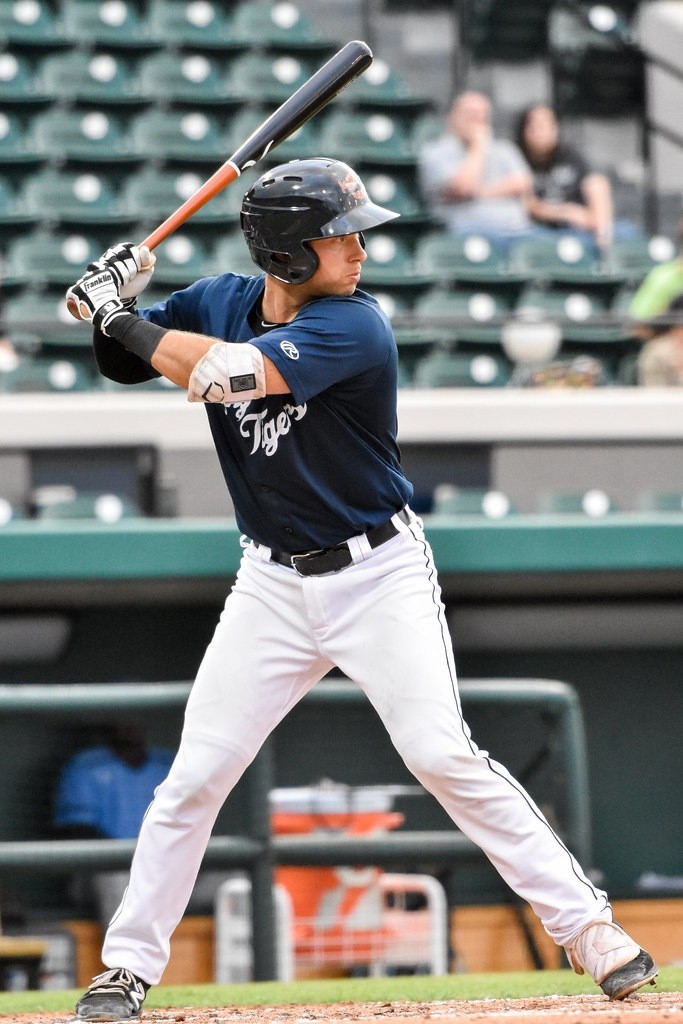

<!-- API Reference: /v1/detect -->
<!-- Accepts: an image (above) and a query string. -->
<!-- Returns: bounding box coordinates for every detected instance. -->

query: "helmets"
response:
[240,157,400,285]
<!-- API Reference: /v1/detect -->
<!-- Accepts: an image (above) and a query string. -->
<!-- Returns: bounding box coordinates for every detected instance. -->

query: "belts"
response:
[253,507,412,578]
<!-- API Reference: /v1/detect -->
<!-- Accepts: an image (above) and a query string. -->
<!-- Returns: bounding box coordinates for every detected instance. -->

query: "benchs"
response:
[2,1,660,399]
[0,904,217,998]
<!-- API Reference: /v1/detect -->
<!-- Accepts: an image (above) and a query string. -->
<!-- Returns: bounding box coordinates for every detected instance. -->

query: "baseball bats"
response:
[64,37,375,323]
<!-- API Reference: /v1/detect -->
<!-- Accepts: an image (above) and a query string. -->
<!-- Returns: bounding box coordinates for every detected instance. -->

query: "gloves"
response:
[99,242,156,309]
[66,262,131,337]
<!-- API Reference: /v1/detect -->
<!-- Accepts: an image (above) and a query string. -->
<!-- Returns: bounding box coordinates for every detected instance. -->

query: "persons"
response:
[64,154,661,1024]
[414,86,614,260]
[56,707,253,970]
[625,220,683,387]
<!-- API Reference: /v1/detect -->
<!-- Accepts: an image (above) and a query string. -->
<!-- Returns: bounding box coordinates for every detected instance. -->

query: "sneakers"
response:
[74,968,152,1022]
[571,920,659,1002]
